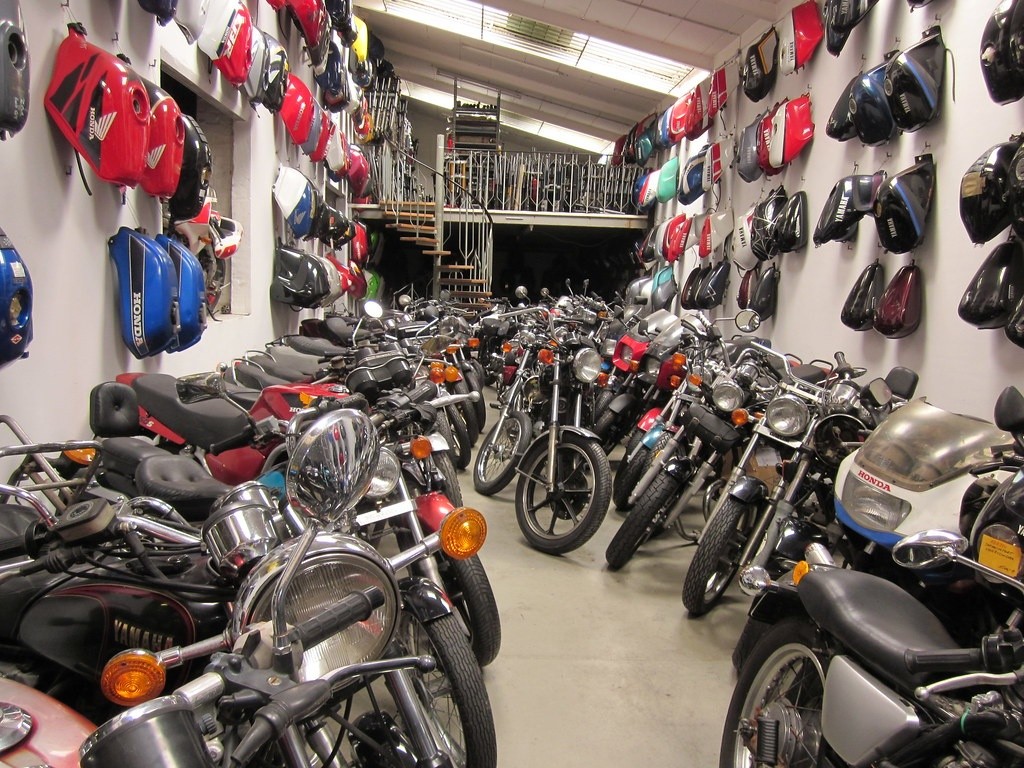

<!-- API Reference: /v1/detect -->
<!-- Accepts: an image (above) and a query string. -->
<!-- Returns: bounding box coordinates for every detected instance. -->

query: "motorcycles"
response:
[0,284,1023,768]
